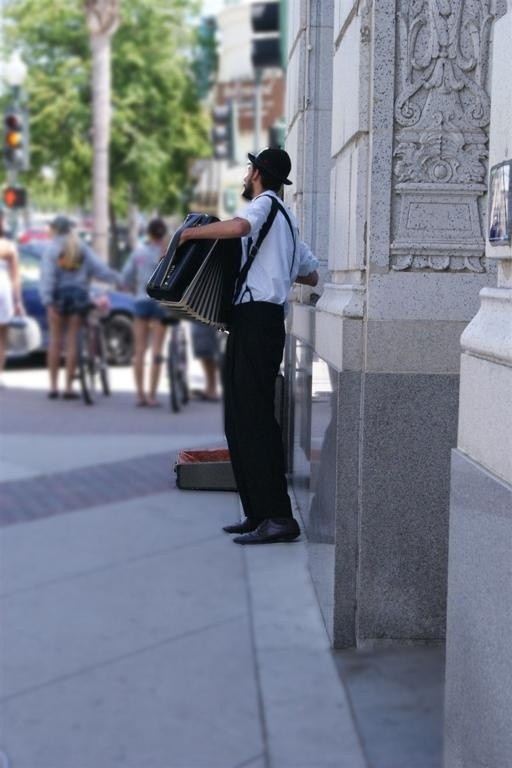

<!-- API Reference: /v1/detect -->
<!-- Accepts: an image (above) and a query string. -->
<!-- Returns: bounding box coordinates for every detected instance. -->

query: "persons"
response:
[113,218,171,409]
[0,206,27,390]
[175,145,321,548]
[190,318,222,405]
[37,215,120,403]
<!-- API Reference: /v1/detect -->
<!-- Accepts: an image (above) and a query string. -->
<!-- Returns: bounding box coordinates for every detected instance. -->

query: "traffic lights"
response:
[211,96,243,168]
[2,107,31,210]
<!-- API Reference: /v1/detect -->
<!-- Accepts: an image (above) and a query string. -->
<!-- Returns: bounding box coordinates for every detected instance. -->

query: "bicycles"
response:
[155,313,190,414]
[70,286,116,405]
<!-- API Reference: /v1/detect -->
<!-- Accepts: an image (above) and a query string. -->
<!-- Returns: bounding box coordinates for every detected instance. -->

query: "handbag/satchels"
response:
[7,314,43,353]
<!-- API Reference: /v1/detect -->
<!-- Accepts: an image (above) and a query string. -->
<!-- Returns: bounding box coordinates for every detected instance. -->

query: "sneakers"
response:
[192,388,218,402]
[134,399,162,408]
[47,390,80,401]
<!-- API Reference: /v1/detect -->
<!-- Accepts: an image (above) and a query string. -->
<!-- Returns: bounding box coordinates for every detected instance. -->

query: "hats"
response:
[247,148,293,186]
[47,216,75,229]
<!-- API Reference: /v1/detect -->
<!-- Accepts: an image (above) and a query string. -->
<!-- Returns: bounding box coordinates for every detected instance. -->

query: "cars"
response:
[13,242,139,366]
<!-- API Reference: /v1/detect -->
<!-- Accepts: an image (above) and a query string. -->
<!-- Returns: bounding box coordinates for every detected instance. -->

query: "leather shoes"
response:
[223,516,266,533]
[233,519,301,545]
